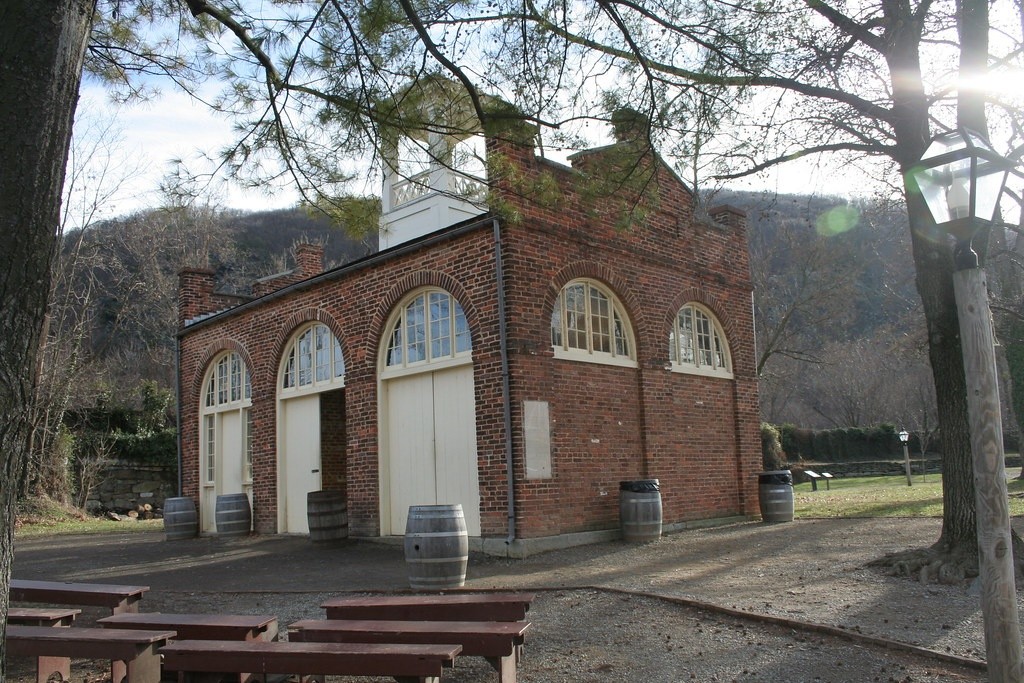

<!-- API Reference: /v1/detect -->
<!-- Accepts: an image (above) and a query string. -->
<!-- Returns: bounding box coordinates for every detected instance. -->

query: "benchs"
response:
[6,579,535,683]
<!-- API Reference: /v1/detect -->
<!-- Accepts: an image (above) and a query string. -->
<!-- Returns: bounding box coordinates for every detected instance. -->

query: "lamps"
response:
[898,427,910,446]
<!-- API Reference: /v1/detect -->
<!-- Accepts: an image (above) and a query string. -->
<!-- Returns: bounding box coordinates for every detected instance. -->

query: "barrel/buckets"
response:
[215,493,251,536]
[163,497,198,539]
[619,478,663,544]
[307,491,348,542]
[404,504,469,590]
[757,469,795,524]
